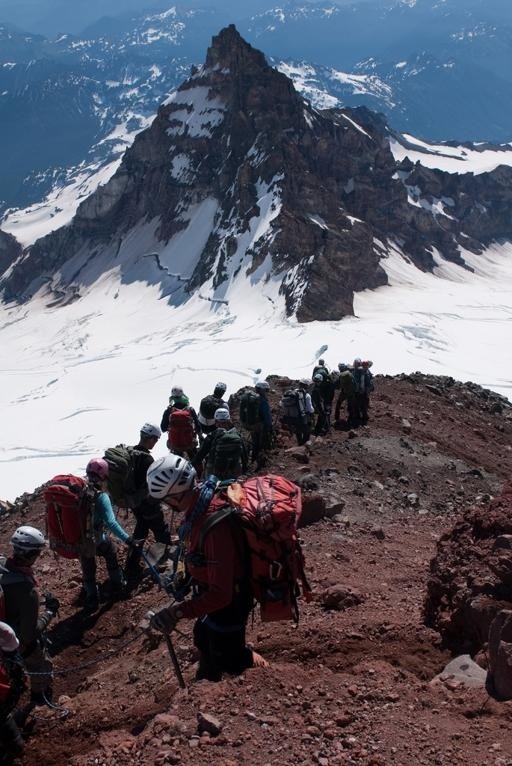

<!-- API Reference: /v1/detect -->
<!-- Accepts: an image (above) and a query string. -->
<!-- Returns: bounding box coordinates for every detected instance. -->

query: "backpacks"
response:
[211,428,242,478]
[282,389,308,417]
[44,475,102,559]
[197,475,303,621]
[168,406,194,450]
[199,396,223,420]
[240,391,265,432]
[104,444,149,498]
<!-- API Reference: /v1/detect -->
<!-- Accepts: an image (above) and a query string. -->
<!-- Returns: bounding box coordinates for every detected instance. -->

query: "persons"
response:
[0,526,60,758]
[161,357,374,481]
[78,422,171,602]
[145,453,253,682]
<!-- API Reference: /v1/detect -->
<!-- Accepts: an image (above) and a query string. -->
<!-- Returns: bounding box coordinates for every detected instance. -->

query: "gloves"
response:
[148,608,177,634]
[45,595,58,610]
[127,538,146,547]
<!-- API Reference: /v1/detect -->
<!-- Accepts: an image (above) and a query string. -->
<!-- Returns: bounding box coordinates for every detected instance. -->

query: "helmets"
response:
[215,408,230,420]
[141,423,161,438]
[85,459,109,476]
[172,386,182,396]
[215,382,226,391]
[146,456,197,500]
[0,622,19,652]
[299,378,309,385]
[11,527,46,550]
[257,381,270,389]
